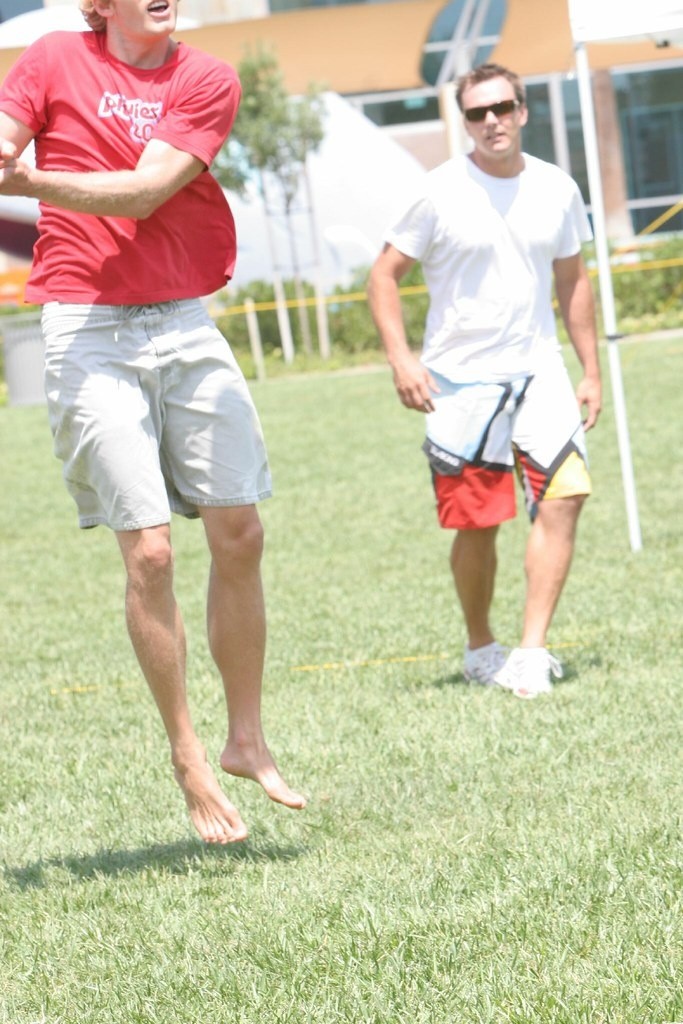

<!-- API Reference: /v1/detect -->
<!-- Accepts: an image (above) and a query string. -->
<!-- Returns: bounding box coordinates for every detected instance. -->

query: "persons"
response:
[367,66,601,700]
[1,0,307,845]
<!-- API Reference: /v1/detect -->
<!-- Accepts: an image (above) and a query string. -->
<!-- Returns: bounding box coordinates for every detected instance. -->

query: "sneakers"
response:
[461,641,506,687]
[494,647,563,700]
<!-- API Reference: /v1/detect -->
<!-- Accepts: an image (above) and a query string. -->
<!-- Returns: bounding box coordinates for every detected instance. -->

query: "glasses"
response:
[465,99,520,123]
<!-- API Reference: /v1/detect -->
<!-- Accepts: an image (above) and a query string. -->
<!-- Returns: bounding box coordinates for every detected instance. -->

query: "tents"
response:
[569,0,682,551]
